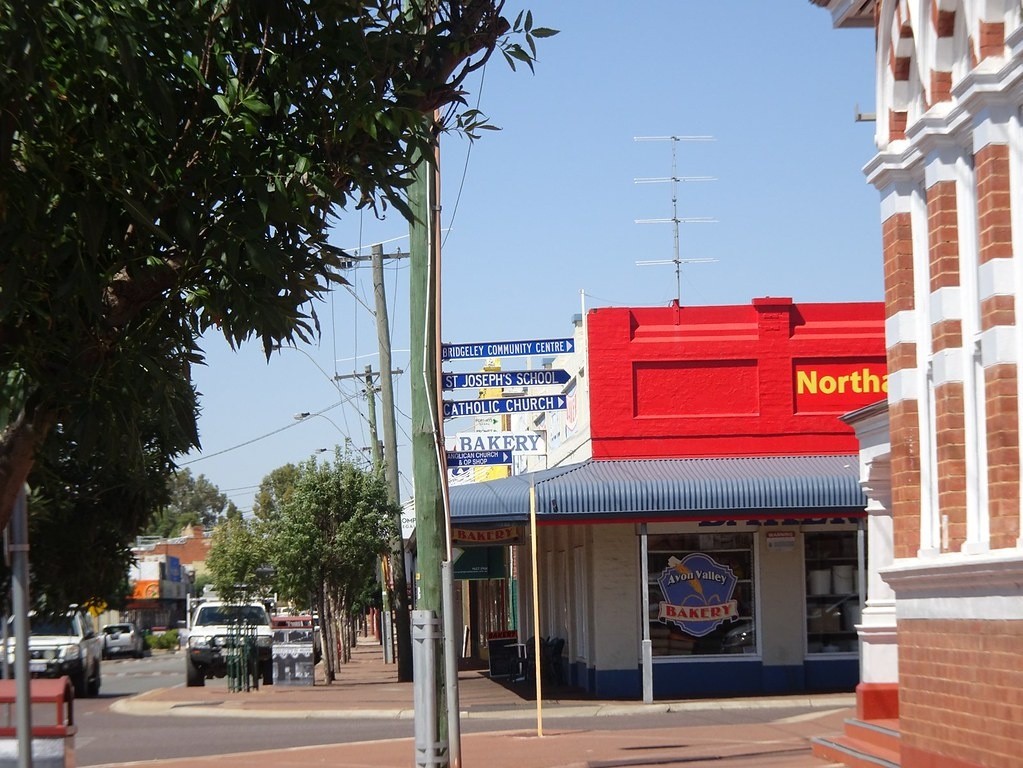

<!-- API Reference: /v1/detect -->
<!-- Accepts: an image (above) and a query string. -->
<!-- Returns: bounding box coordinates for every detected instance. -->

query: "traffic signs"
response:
[445,448,513,469]
[442,369,570,390]
[444,339,575,360]
[441,394,569,418]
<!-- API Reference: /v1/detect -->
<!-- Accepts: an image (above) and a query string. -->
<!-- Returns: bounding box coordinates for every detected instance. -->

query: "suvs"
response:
[186,600,275,688]
[1,597,104,702]
[96,622,151,661]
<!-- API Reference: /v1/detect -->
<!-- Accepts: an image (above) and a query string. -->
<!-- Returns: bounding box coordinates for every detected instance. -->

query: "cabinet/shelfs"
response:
[648,531,867,657]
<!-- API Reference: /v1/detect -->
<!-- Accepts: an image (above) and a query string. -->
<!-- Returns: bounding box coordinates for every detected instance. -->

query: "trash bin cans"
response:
[1,676,79,768]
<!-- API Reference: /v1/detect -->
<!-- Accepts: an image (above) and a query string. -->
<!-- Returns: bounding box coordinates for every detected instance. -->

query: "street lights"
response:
[262,345,399,664]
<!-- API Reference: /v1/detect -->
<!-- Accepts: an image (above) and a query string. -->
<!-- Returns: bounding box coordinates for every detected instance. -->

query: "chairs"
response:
[509,635,547,686]
